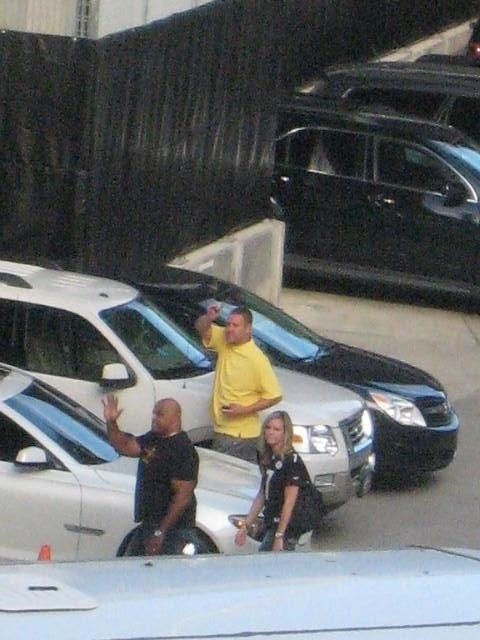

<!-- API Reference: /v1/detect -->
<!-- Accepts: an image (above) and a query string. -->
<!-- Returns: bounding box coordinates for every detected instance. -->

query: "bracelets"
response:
[241,522,251,531]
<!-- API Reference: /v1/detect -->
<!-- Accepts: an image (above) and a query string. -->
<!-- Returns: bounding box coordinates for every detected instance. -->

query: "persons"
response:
[101,394,200,556]
[194,301,283,460]
[234,410,323,552]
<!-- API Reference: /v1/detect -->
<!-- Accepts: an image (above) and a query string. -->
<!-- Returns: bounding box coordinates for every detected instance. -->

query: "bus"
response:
[0,542,480,639]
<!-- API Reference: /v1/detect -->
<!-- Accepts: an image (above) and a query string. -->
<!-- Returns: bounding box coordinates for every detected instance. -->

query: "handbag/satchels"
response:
[280,481,327,536]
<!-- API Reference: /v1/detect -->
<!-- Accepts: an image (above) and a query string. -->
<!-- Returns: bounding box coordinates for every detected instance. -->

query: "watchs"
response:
[274,532,284,539]
[151,529,166,539]
